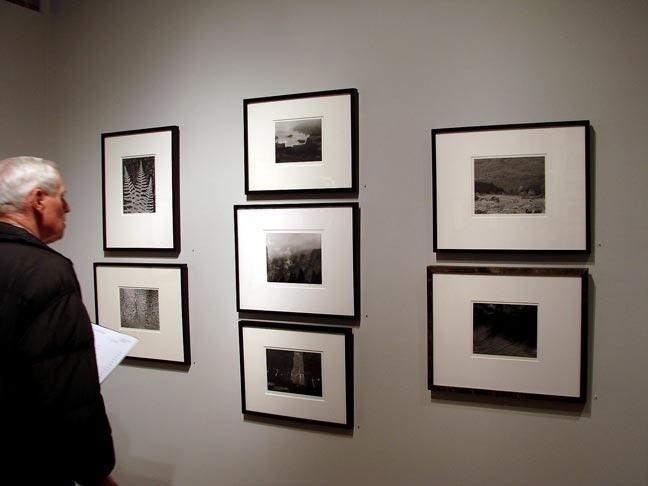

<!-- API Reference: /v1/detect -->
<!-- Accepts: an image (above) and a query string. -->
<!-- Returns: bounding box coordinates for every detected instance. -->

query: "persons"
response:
[0,155,118,486]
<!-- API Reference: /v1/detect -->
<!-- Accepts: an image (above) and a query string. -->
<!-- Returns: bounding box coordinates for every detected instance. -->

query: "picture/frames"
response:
[98,125,184,253]
[240,86,360,197]
[423,263,588,408]
[238,320,354,431]
[230,203,362,323]
[91,261,192,368]
[430,119,595,257]
